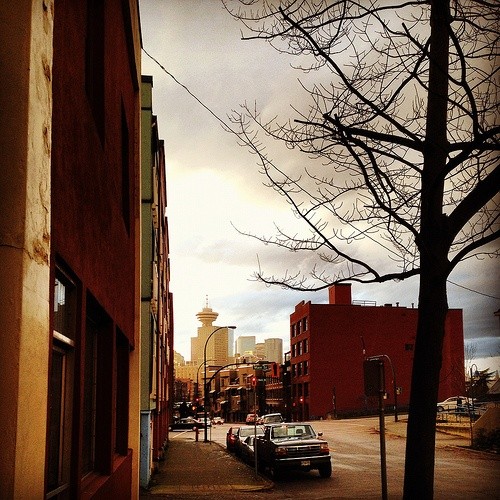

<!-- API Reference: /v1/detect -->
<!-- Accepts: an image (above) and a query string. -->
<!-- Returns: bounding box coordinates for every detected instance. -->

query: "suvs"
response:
[437,396,474,412]
[246,413,286,425]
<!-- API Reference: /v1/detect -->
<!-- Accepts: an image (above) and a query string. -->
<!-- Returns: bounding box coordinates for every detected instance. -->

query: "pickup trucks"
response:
[253,424,332,482]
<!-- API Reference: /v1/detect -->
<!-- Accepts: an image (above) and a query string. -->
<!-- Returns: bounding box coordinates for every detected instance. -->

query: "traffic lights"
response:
[192,405,196,411]
[300,397,303,403]
[252,376,256,386]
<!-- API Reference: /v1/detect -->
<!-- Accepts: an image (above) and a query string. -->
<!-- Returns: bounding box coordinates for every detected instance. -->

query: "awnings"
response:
[209,389,215,393]
[235,377,239,380]
[225,387,230,391]
[238,387,242,390]
[247,374,253,377]
[220,401,228,404]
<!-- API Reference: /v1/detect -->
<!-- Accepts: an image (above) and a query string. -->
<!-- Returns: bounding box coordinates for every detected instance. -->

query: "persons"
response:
[457,395,462,406]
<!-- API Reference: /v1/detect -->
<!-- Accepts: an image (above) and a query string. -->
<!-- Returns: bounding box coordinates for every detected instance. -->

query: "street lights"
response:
[470,364,479,415]
[204,325,269,442]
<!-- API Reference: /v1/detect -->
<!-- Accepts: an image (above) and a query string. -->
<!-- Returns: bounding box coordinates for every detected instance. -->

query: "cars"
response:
[201,417,224,427]
[168,418,205,432]
[226,426,265,464]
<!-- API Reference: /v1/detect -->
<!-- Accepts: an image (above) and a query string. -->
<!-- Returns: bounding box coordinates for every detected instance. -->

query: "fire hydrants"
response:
[193,427,199,441]
